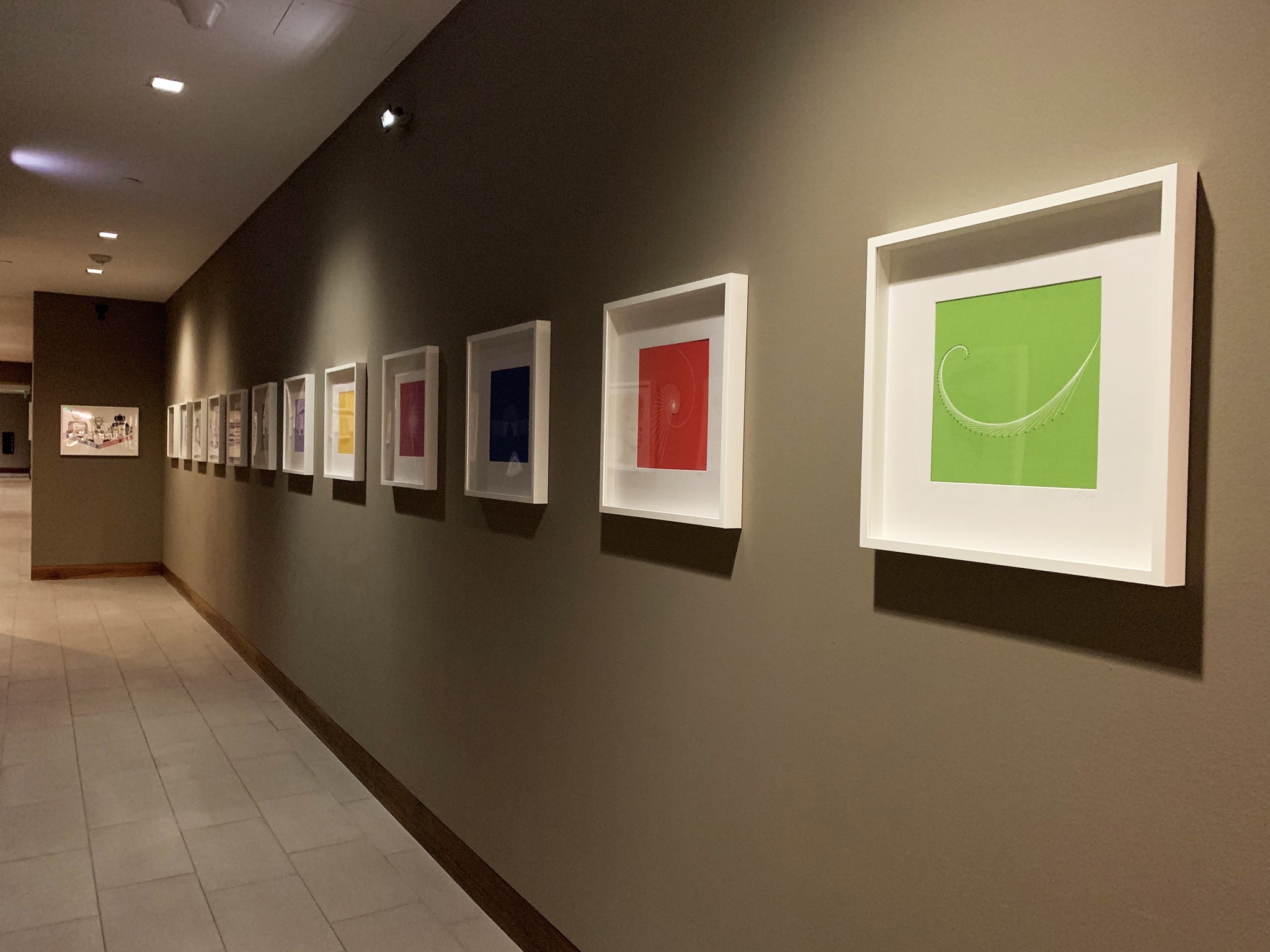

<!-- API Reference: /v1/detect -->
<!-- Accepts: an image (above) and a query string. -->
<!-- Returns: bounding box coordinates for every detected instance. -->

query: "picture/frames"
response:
[167,395,225,463]
[600,272,749,528]
[251,382,277,470]
[380,346,438,490]
[282,374,315,475]
[859,162,1197,587]
[226,389,248,467]
[324,362,366,481]
[60,405,138,456]
[464,320,551,504]
